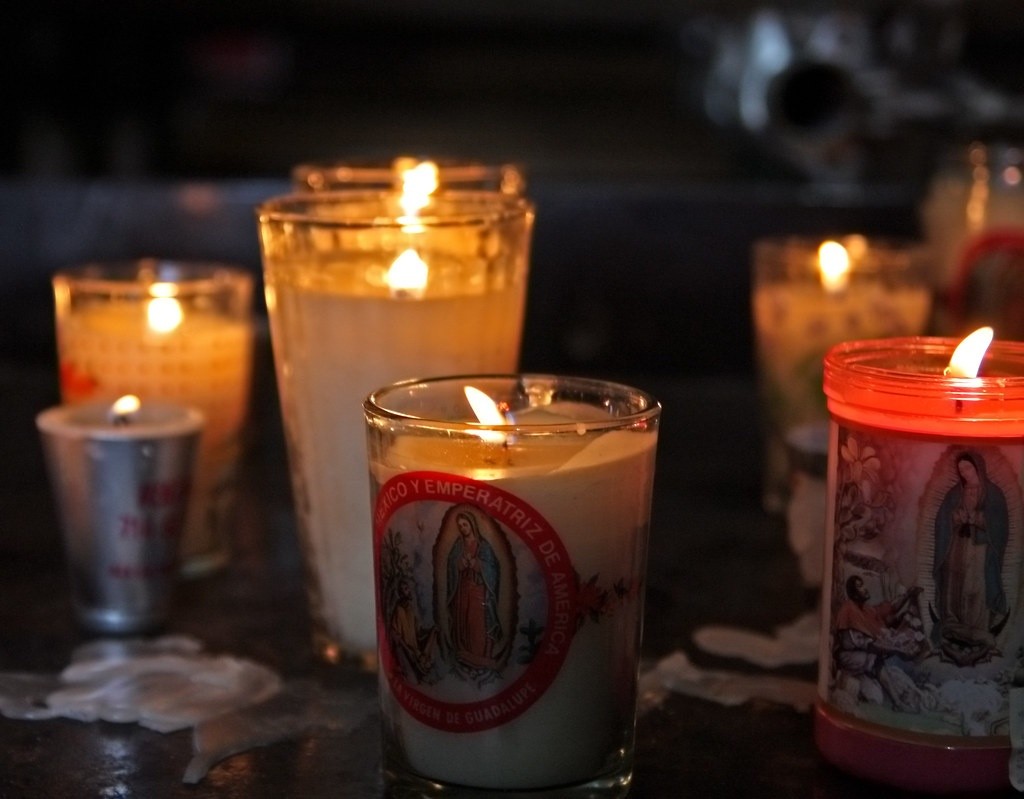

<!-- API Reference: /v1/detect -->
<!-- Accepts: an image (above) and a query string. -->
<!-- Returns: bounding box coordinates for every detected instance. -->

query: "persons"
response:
[732,63,922,250]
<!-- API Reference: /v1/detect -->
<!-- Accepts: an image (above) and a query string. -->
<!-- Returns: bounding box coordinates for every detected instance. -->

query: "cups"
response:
[294,162,526,198]
[257,190,535,670]
[754,241,933,509]
[817,337,1024,796]
[38,405,205,634]
[923,148,1024,342]
[55,261,255,577]
[360,374,661,799]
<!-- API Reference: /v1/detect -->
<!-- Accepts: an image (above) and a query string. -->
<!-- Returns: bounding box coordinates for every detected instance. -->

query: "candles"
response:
[366,406,655,787]
[259,248,525,646]
[756,239,1024,787]
[37,298,250,633]
